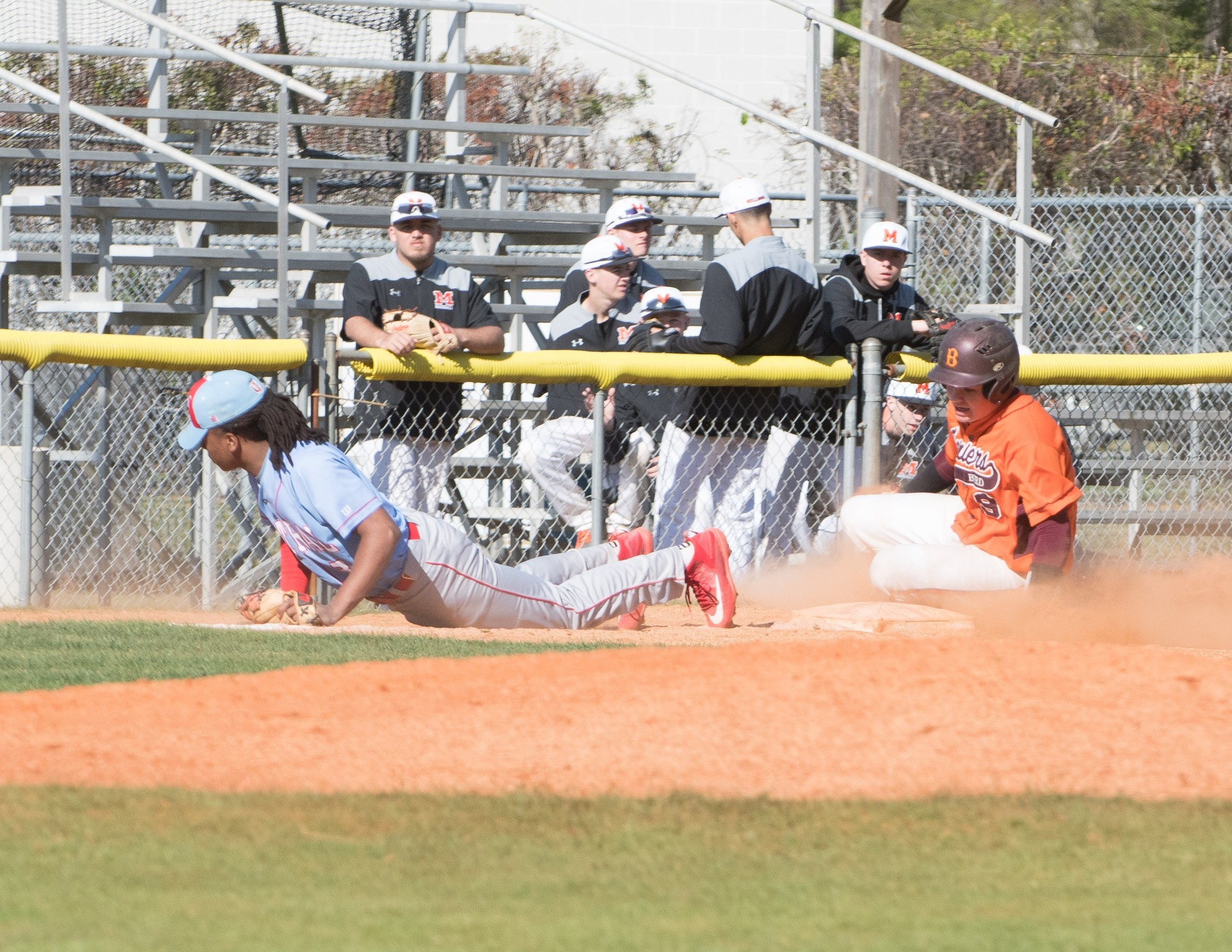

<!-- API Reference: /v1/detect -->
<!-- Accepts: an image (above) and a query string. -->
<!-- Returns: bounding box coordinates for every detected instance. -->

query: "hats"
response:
[861,221,913,254]
[886,378,940,406]
[604,198,664,231]
[177,369,266,451]
[580,234,646,271]
[639,286,689,318]
[390,190,442,225]
[713,178,770,219]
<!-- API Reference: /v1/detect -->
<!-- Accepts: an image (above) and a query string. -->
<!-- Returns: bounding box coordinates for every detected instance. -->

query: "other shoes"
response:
[575,530,592,548]
[607,532,621,540]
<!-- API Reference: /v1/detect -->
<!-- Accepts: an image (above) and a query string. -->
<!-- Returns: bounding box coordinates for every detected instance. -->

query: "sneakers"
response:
[609,527,653,630]
[686,530,737,628]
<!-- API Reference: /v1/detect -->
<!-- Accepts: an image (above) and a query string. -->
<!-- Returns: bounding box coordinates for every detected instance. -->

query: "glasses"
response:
[584,247,633,265]
[606,207,652,227]
[391,203,437,214]
[1126,333,1144,346]
[639,298,685,313]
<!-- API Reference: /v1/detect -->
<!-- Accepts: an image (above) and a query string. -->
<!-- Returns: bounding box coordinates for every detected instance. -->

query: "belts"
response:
[365,522,418,605]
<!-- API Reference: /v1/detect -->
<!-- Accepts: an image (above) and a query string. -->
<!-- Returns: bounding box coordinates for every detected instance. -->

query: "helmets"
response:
[928,317,1019,404]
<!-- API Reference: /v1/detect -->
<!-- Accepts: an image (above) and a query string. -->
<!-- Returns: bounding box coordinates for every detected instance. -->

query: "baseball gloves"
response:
[382,308,460,355]
[623,320,680,354]
[915,307,960,352]
[233,589,318,625]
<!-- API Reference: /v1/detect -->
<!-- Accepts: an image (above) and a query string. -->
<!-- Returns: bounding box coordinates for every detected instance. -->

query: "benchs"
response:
[0,186,1020,524]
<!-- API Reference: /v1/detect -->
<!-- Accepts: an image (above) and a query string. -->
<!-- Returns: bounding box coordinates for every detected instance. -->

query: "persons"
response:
[341,191,505,516]
[178,369,739,629]
[519,181,960,577]
[813,317,1082,593]
